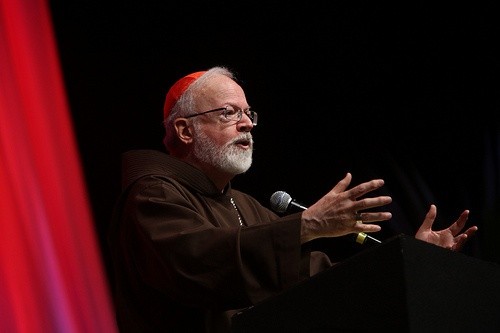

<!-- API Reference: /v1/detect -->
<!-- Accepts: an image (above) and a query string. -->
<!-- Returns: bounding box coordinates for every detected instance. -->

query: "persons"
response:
[93,65,478,332]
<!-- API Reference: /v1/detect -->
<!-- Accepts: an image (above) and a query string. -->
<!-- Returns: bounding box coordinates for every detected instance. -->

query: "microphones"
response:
[269,190,384,248]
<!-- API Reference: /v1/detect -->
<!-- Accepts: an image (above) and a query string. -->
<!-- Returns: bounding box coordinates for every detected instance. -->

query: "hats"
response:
[163,67,222,159]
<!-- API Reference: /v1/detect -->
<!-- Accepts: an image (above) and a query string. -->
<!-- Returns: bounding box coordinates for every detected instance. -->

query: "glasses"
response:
[186,105,257,123]
